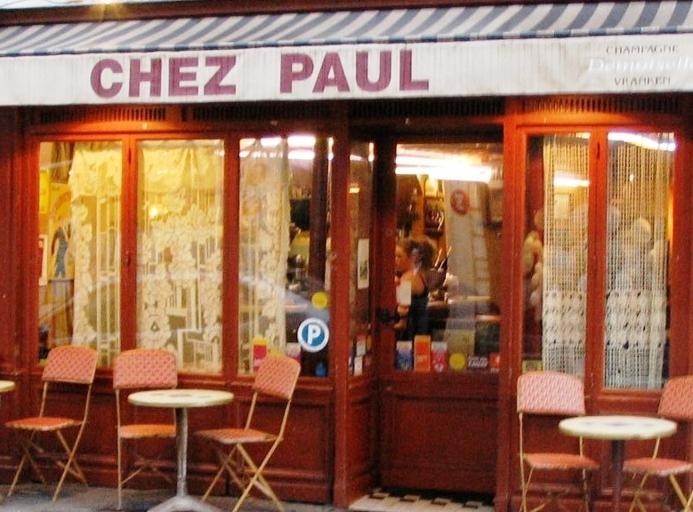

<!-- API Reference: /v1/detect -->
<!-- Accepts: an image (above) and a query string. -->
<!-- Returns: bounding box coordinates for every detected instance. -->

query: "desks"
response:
[560,414,678,512]
[128,389,235,512]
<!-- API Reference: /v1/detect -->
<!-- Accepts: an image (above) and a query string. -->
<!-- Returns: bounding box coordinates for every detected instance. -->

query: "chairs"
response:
[611,375,693,512]
[6,346,98,503]
[517,371,600,512]
[193,354,301,512]
[112,348,178,511]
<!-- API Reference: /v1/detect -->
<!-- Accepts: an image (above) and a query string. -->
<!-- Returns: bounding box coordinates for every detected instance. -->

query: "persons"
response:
[393,234,439,342]
[51,225,72,279]
